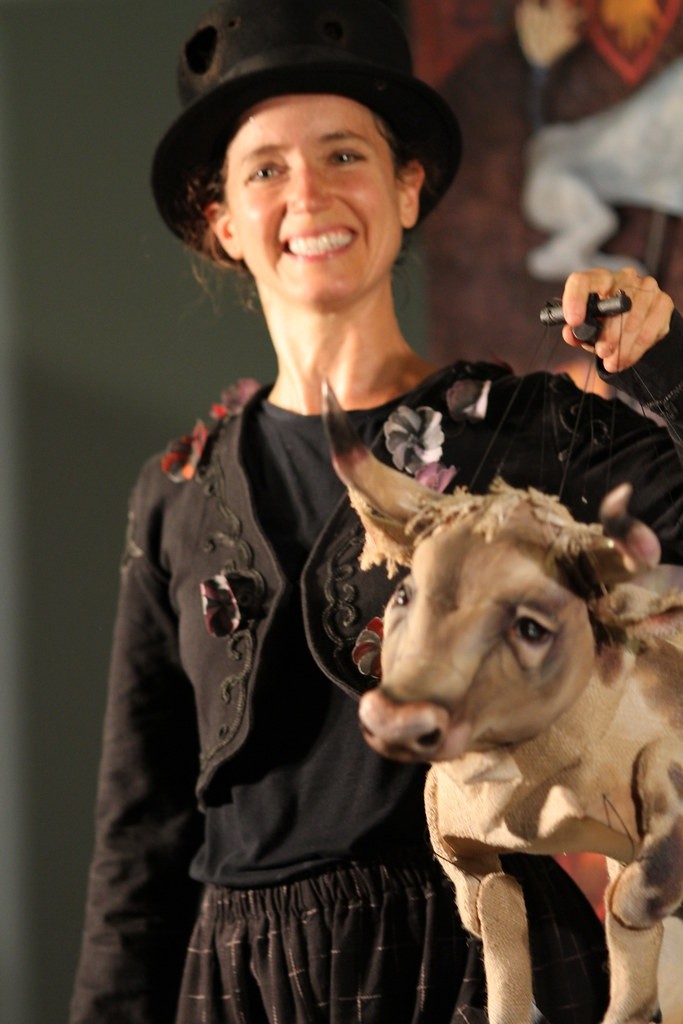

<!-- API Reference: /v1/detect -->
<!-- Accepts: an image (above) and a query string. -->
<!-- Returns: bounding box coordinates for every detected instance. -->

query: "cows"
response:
[312,377,683,1024]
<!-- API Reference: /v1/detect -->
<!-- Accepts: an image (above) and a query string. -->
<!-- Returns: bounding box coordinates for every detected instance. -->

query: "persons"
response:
[68,0,683,1024]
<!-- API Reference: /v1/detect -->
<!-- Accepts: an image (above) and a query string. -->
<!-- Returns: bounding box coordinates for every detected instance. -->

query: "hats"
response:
[152,0,464,267]
